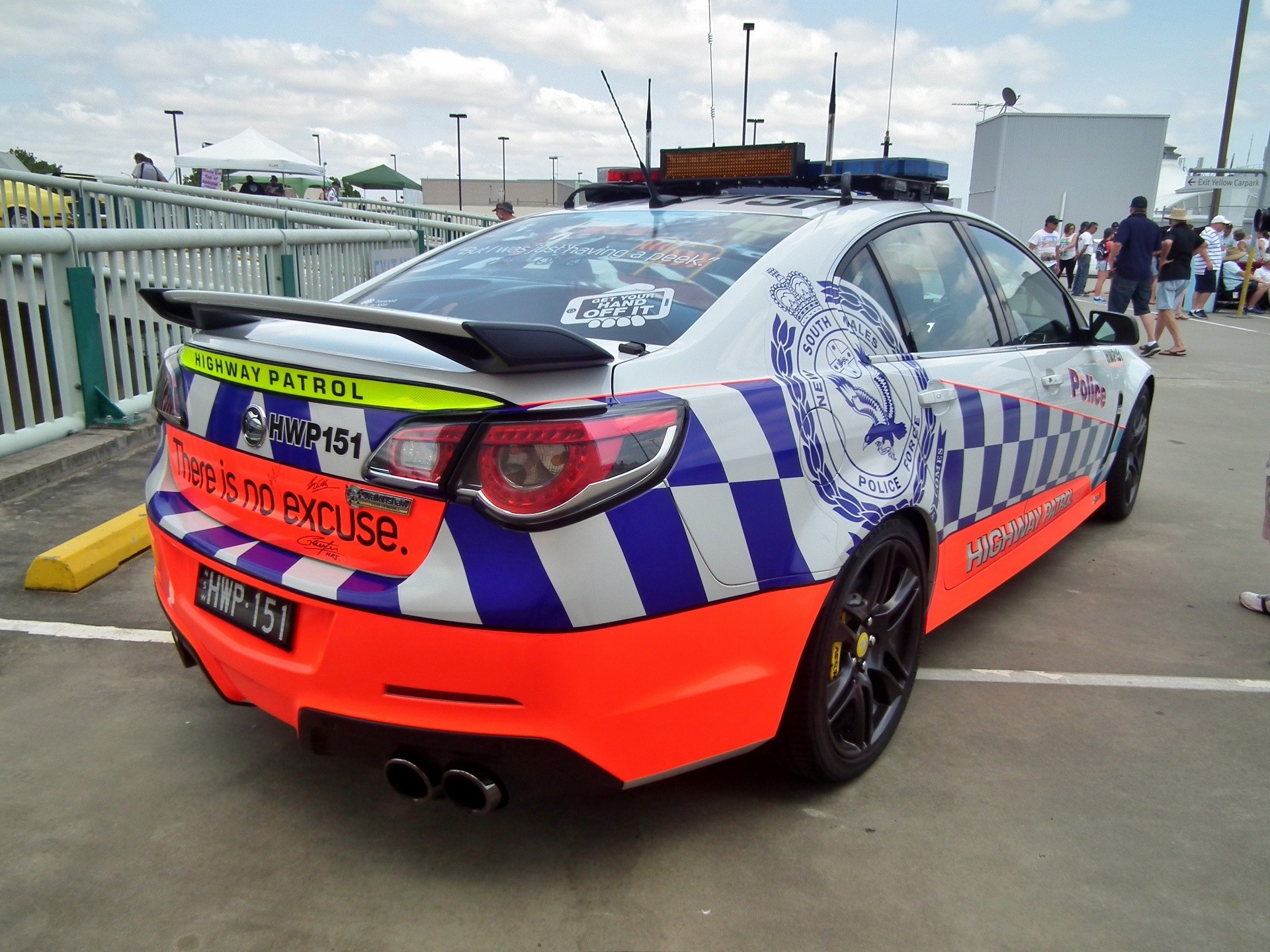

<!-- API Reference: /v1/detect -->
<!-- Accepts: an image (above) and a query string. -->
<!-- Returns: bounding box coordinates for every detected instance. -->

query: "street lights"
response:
[449,114,467,211]
[390,154,398,203]
[164,110,184,184]
[747,119,764,145]
[312,134,321,166]
[498,137,509,201]
[549,157,558,205]
[742,23,755,146]
[578,172,583,182]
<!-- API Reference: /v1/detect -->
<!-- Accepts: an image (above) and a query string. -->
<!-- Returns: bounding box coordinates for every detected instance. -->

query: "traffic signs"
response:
[1185,175,1263,189]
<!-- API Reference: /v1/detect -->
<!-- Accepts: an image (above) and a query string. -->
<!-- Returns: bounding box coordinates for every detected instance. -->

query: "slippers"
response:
[1159,350,1186,356]
[1139,345,1146,350]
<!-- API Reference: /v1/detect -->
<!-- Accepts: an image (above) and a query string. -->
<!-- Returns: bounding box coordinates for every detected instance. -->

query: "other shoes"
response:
[1072,293,1089,297]
[1155,314,1159,320]
[1175,313,1189,319]
[1093,297,1107,303]
[1067,288,1072,292]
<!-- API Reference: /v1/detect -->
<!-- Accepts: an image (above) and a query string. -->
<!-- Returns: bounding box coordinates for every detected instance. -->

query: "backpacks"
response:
[1095,239,1111,260]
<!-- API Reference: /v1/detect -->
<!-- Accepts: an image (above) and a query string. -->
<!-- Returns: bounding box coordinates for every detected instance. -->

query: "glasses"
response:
[1053,222,1058,225]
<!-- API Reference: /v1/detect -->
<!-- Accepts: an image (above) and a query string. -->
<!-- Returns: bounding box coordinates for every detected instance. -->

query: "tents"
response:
[173,126,326,229]
[221,170,334,217]
[341,164,423,223]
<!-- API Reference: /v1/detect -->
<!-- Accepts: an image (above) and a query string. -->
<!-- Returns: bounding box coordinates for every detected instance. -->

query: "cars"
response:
[137,142,1156,815]
[0,152,107,229]
[1061,239,1103,276]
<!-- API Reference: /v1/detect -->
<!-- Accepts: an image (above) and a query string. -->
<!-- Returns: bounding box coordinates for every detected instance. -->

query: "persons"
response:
[1218,223,1237,258]
[1107,196,1162,357]
[1223,247,1269,314]
[492,201,516,222]
[239,175,265,205]
[229,187,238,202]
[327,181,341,202]
[132,153,169,229]
[381,196,388,201]
[1139,209,1207,356]
[1188,215,1232,319]
[1028,215,1063,279]
[1232,229,1261,261]
[1239,456,1270,616]
[1257,231,1270,262]
[1059,223,1077,292]
[1072,221,1192,320]
[264,175,283,196]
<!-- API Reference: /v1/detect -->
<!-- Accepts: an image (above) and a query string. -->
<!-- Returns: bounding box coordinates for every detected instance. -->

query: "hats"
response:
[1226,224,1233,229]
[1210,215,1230,224]
[1130,196,1147,207]
[1163,208,1188,220]
[492,202,514,214]
[1111,222,1118,228]
[269,175,278,181]
[1046,215,1063,222]
[1222,247,1247,261]
[332,181,342,188]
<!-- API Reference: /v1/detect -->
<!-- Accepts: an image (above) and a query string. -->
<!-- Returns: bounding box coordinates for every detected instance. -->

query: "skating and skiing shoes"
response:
[1244,306,1265,315]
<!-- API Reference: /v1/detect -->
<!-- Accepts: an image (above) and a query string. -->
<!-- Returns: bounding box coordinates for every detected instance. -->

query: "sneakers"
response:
[1192,310,1209,319]
[1187,310,1197,318]
[1239,592,1270,615]
[1140,342,1160,357]
